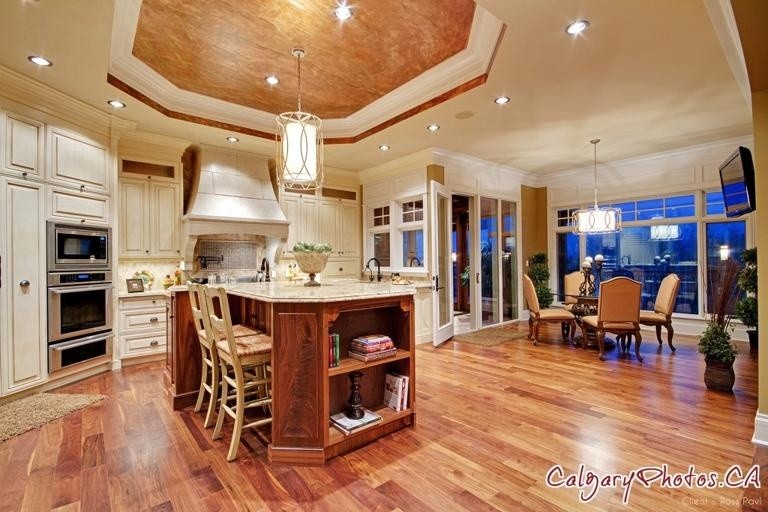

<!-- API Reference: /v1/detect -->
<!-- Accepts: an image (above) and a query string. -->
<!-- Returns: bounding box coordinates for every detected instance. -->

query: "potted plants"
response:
[697,314,739,392]
[290,240,333,286]
[735,245,759,350]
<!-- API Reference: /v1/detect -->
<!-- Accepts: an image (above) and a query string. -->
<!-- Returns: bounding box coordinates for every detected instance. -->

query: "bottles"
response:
[174,265,181,286]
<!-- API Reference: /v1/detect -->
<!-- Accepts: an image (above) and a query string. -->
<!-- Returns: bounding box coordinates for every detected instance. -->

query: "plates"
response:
[132,269,154,289]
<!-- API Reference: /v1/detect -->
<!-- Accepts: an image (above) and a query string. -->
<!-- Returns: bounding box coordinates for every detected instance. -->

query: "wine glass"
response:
[288,250,332,287]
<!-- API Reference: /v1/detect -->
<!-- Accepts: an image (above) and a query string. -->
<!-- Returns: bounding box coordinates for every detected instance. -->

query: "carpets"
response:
[451,326,530,347]
[0,393,110,443]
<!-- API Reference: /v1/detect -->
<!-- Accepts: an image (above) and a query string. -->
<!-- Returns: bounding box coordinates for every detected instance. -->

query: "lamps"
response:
[579,254,606,297]
[571,139,624,235]
[274,49,325,190]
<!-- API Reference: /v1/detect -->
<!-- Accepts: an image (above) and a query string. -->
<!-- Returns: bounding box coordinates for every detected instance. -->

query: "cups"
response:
[208,271,238,289]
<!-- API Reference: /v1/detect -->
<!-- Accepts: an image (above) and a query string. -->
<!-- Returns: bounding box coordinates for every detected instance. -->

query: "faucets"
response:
[260,257,271,281]
[410,257,420,267]
[367,258,383,282]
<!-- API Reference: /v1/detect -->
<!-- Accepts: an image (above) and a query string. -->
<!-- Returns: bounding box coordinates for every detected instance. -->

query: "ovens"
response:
[46,284,114,376]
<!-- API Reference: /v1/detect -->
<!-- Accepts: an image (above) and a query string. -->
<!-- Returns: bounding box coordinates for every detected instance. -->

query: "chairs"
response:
[523,270,682,362]
[186,280,272,462]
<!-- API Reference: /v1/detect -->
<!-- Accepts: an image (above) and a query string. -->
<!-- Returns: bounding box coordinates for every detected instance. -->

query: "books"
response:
[329,406,382,430]
[382,372,409,412]
[346,333,397,363]
[328,332,341,368]
[329,417,384,438]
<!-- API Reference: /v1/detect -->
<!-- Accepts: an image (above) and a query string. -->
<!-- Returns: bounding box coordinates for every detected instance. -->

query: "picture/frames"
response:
[126,279,144,292]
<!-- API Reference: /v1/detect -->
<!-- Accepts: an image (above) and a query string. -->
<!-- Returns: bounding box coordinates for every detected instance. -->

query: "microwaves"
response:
[54,228,108,265]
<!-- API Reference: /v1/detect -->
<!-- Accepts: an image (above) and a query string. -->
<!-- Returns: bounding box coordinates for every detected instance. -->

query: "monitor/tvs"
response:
[717,145,756,219]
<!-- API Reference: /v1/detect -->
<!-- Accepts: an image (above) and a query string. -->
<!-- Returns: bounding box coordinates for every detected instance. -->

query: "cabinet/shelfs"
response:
[263,158,363,280]
[118,293,168,367]
[45,113,114,231]
[0,95,47,404]
[118,151,184,260]
[321,295,418,465]
